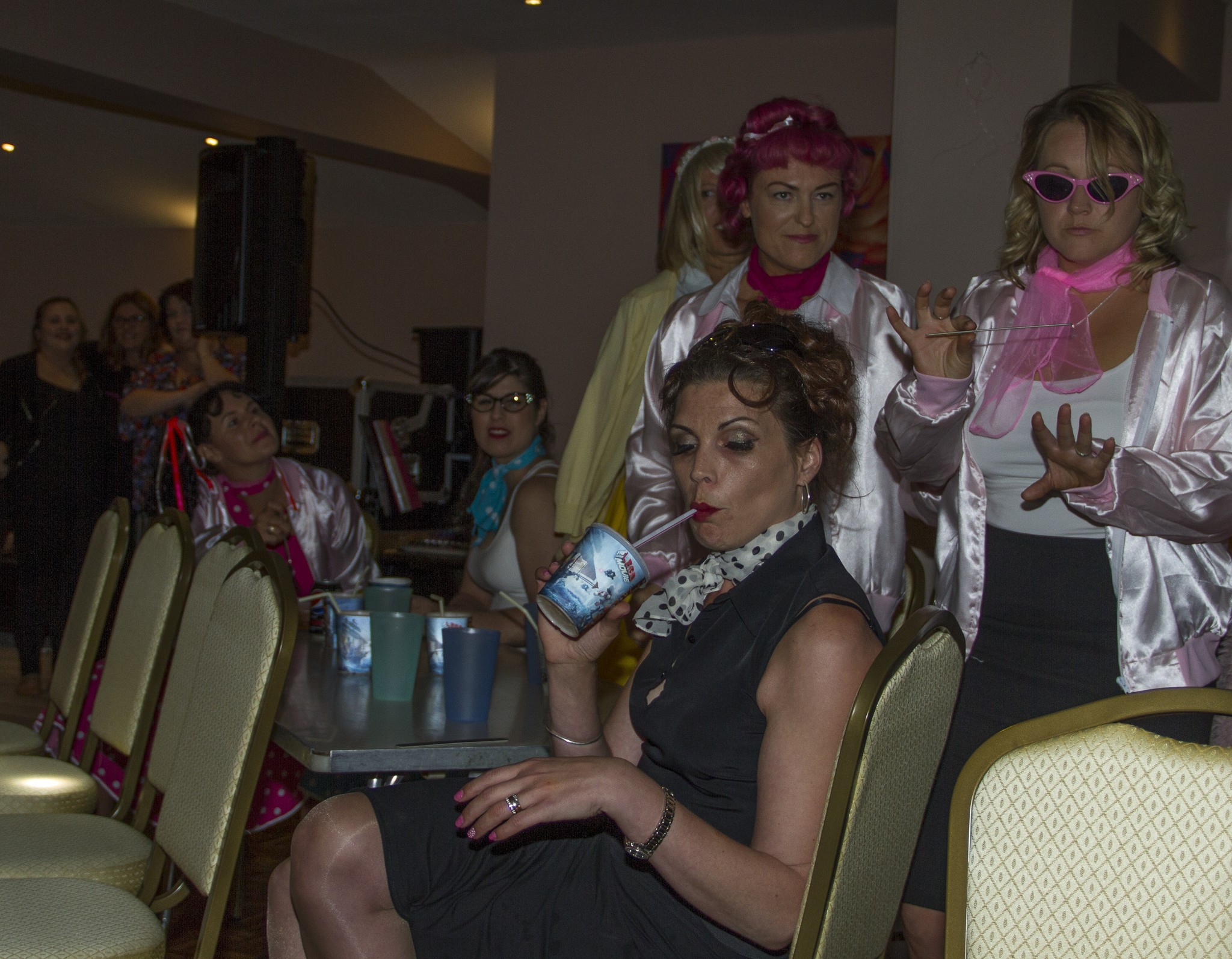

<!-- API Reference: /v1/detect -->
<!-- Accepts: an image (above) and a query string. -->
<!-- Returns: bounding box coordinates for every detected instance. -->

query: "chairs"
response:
[946,687,1232,959]
[789,604,968,959]
[0,495,133,757]
[0,549,301,959]
[1,524,265,898]
[1,505,196,822]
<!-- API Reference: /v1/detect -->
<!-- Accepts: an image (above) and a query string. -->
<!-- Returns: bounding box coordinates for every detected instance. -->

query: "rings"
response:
[1074,447,1092,455]
[504,793,522,814]
[932,312,951,321]
[267,525,276,533]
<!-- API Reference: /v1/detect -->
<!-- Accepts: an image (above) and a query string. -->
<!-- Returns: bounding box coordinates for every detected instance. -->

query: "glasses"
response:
[1022,163,1144,203]
[463,389,536,413]
[113,314,148,325]
[687,323,815,371]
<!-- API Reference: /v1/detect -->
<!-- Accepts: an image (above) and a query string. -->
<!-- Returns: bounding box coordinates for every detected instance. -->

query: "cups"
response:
[426,612,472,676]
[335,610,372,674]
[441,627,501,724]
[536,522,650,638]
[365,577,414,612]
[523,602,549,696]
[370,611,424,702]
[325,591,364,650]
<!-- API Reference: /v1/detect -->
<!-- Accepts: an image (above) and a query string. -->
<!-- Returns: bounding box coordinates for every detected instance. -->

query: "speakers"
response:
[191,135,313,338]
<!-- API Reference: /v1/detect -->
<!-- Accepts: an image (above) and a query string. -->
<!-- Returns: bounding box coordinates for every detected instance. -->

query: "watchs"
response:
[624,787,675,859]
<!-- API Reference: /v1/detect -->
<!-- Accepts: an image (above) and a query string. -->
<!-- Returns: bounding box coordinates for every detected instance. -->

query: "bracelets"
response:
[542,707,605,746]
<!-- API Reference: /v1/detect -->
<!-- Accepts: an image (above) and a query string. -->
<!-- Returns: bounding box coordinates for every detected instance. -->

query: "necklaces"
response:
[1069,284,1119,329]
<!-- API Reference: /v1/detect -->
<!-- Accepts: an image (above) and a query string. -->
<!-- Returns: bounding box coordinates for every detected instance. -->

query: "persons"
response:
[866,74,1232,959]
[0,277,386,838]
[622,95,925,666]
[392,347,563,648]
[263,286,902,958]
[554,130,751,557]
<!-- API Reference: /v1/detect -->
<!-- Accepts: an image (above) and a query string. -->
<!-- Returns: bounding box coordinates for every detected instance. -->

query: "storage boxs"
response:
[280,377,455,510]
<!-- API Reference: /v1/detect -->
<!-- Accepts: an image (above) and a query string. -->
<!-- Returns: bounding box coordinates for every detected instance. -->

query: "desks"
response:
[270,629,629,792]
[374,529,464,593]
